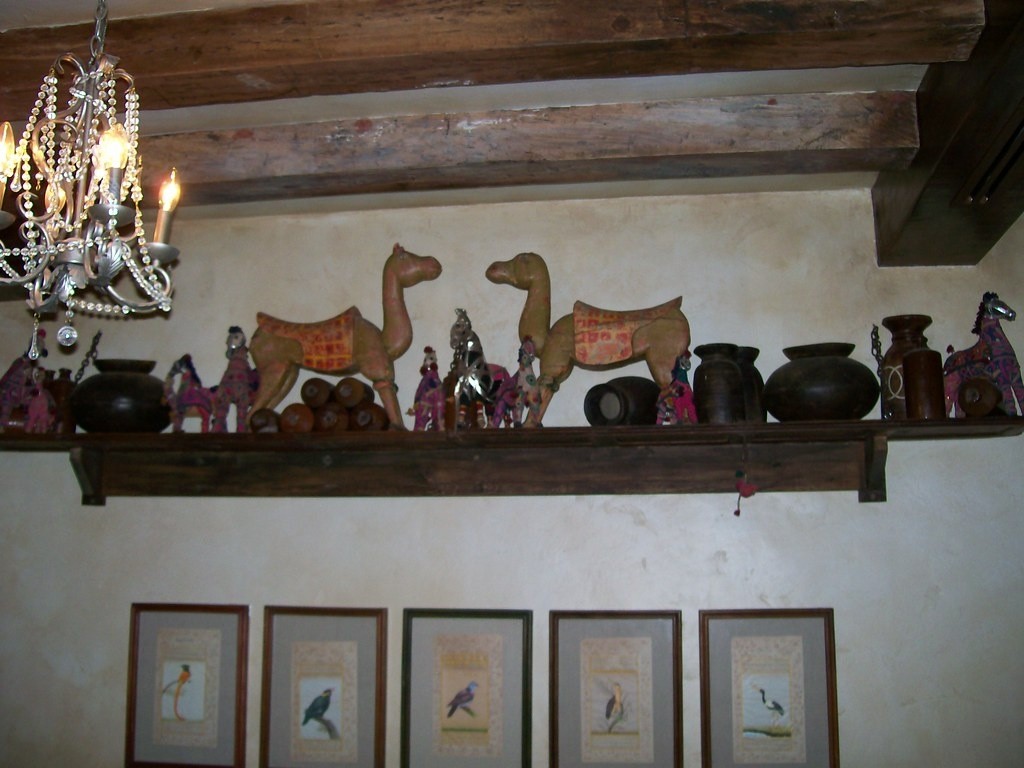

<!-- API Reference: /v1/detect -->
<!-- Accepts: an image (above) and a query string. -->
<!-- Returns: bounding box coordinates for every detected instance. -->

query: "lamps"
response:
[0,0,181,358]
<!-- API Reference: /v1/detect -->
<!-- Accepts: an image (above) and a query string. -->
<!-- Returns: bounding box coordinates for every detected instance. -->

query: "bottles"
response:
[584,377,662,426]
[69,358,170,432]
[764,343,880,422]
[881,314,932,419]
[694,343,768,425]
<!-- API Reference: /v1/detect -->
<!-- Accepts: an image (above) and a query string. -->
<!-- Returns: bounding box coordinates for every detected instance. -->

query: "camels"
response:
[484,250,691,428]
[242,240,444,433]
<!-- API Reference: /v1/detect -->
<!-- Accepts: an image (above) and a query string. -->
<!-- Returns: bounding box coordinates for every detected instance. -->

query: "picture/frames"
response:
[123,603,248,768]
[257,603,387,768]
[697,608,841,768]
[548,609,684,768]
[399,607,534,768]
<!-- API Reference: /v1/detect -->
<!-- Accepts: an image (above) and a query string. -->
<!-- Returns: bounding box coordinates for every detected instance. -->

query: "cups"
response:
[902,337,945,419]
